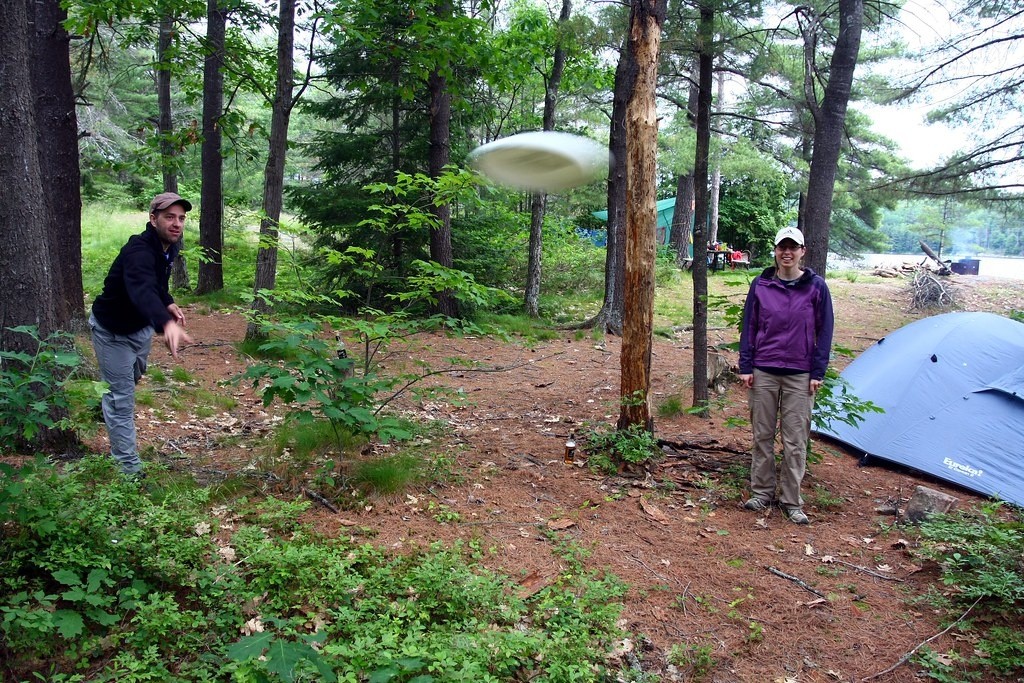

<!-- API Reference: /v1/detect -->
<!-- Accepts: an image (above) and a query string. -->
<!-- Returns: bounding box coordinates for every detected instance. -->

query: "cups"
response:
[718,242,723,251]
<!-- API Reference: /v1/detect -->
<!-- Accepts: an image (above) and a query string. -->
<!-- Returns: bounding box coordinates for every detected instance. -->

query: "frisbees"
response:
[470,131,616,193]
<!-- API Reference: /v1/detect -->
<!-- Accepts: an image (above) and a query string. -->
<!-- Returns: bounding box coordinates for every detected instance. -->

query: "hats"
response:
[774,227,806,247]
[150,192,192,220]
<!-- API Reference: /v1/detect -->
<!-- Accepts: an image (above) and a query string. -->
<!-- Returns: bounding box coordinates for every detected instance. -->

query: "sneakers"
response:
[744,498,767,510]
[780,502,809,524]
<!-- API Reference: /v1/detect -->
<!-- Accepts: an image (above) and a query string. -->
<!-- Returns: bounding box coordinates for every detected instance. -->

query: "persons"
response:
[88,191,197,477]
[737,226,835,524]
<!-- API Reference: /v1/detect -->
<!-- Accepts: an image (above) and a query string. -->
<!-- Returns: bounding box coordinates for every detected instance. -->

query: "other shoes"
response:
[89,404,106,424]
[123,474,147,494]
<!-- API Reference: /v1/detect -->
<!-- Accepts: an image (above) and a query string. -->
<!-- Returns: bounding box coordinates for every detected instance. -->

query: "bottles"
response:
[564,433,576,467]
[335,336,347,358]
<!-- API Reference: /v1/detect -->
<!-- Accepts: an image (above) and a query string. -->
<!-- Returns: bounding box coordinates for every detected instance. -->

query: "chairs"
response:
[681,249,751,274]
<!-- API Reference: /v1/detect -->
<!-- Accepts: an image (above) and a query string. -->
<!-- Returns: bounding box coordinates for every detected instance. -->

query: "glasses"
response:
[775,244,801,251]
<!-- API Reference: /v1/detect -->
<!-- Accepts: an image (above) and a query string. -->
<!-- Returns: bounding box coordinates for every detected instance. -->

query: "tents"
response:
[592,195,711,270]
[813,310,1023,508]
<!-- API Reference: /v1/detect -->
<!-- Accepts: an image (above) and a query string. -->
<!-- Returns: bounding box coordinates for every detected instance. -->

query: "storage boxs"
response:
[951,259,981,275]
